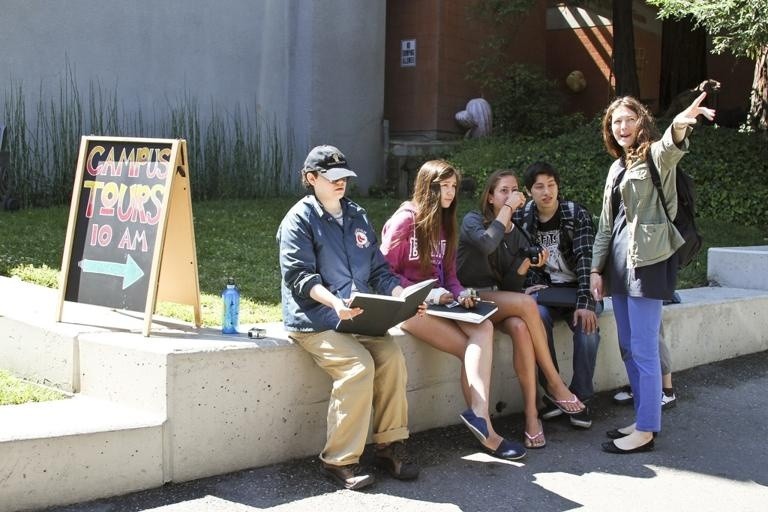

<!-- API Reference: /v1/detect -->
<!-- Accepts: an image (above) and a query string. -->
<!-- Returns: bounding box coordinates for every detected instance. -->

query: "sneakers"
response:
[321,463,375,490]
[479,438,527,460]
[459,407,489,441]
[661,389,676,411]
[613,385,634,405]
[374,440,419,481]
[543,385,593,429]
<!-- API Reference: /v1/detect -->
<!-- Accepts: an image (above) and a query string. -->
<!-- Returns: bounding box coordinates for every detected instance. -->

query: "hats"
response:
[304,145,358,182]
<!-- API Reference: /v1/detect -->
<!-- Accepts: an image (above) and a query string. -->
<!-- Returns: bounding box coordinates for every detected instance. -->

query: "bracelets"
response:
[674,126,686,130]
[503,204,512,212]
[590,272,600,275]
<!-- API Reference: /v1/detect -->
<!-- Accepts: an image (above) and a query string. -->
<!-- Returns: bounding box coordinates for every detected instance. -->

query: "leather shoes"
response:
[601,428,655,454]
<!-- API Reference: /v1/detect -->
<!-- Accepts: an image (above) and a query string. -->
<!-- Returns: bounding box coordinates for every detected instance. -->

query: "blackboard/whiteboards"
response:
[64,141,173,314]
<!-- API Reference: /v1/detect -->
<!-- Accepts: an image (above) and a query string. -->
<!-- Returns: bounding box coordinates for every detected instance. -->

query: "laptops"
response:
[536,286,580,308]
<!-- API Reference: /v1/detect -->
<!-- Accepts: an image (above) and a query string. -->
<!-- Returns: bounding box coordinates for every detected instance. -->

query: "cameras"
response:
[459,287,480,302]
[523,244,543,264]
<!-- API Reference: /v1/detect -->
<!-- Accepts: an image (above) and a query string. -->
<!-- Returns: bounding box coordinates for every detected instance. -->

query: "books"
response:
[426,299,499,325]
[336,276,439,337]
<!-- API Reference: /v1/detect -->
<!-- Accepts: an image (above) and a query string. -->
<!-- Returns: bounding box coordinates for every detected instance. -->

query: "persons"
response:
[456,168,586,450]
[510,161,600,429]
[615,166,696,413]
[590,92,716,454]
[380,160,528,461]
[277,144,420,490]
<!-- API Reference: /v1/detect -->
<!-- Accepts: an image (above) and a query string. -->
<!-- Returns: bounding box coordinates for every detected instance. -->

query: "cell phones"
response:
[248,327,267,339]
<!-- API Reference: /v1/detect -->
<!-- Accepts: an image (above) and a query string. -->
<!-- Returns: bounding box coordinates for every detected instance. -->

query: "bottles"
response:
[222,283,239,334]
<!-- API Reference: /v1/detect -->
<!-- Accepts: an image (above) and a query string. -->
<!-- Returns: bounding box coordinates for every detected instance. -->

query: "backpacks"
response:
[646,141,702,270]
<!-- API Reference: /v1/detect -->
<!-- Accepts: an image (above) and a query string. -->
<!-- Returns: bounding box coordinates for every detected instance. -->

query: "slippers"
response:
[525,419,546,449]
